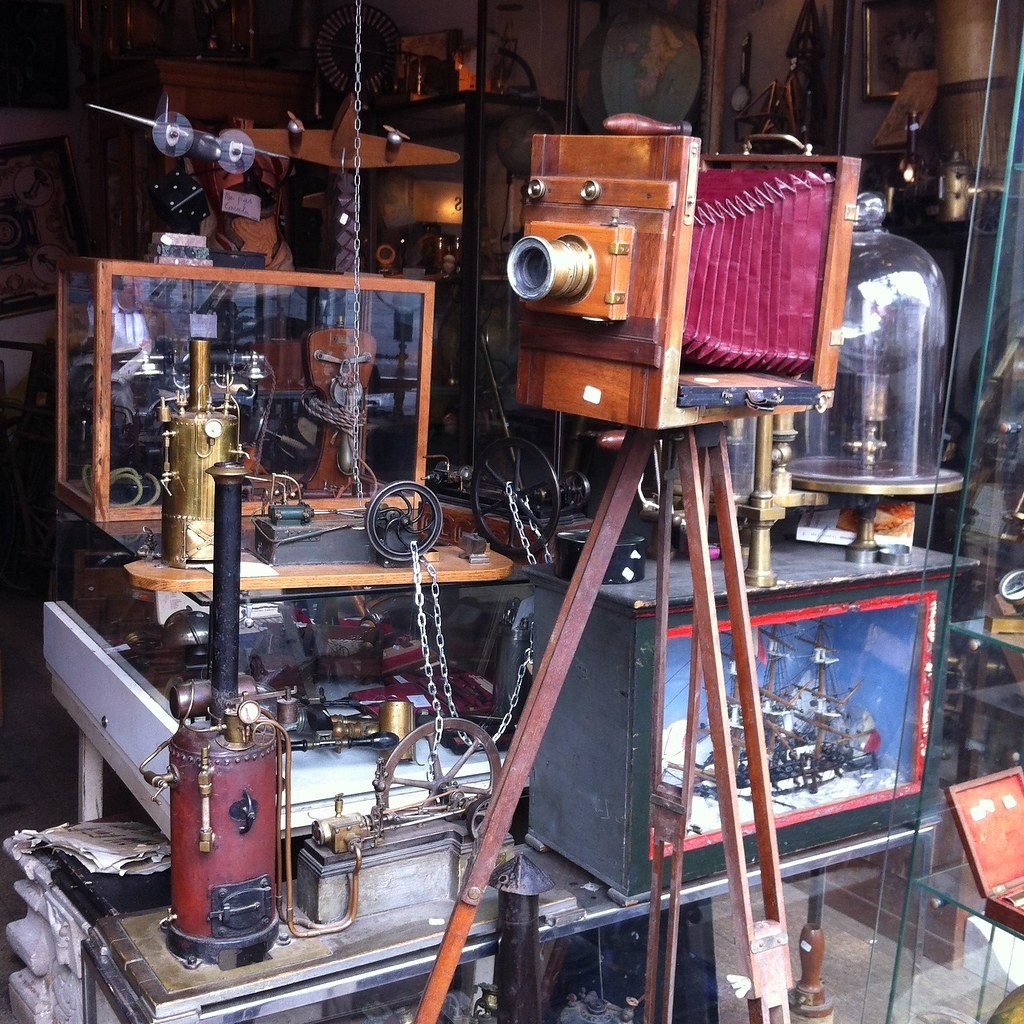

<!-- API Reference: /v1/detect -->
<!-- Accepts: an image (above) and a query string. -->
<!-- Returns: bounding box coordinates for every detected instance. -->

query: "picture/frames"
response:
[108,0,254,64]
[0,135,97,321]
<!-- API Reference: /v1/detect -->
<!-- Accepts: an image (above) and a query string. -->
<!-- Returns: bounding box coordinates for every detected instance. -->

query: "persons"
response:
[43,272,181,467]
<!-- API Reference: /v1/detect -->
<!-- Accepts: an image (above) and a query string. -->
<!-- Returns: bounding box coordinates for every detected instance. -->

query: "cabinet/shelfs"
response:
[312,91,563,287]
[76,58,339,260]
[861,0,1024,1024]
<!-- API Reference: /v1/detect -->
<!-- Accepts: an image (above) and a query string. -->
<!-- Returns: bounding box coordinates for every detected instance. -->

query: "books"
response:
[796,501,916,552]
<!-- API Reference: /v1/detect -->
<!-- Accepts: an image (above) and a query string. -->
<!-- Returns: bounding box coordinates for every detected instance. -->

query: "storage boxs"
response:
[52,251,435,524]
[943,766,1024,934]
[520,542,982,909]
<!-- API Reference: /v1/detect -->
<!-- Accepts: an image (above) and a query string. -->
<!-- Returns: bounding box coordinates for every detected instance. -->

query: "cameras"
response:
[507,113,862,430]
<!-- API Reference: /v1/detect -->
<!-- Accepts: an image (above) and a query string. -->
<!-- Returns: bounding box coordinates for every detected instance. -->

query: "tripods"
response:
[413,423,796,1024]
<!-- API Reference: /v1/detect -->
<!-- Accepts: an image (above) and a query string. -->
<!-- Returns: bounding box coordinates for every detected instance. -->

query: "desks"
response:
[50,673,491,844]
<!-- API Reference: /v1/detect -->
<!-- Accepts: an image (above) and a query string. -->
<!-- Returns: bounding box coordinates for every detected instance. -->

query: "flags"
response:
[757,630,768,665]
